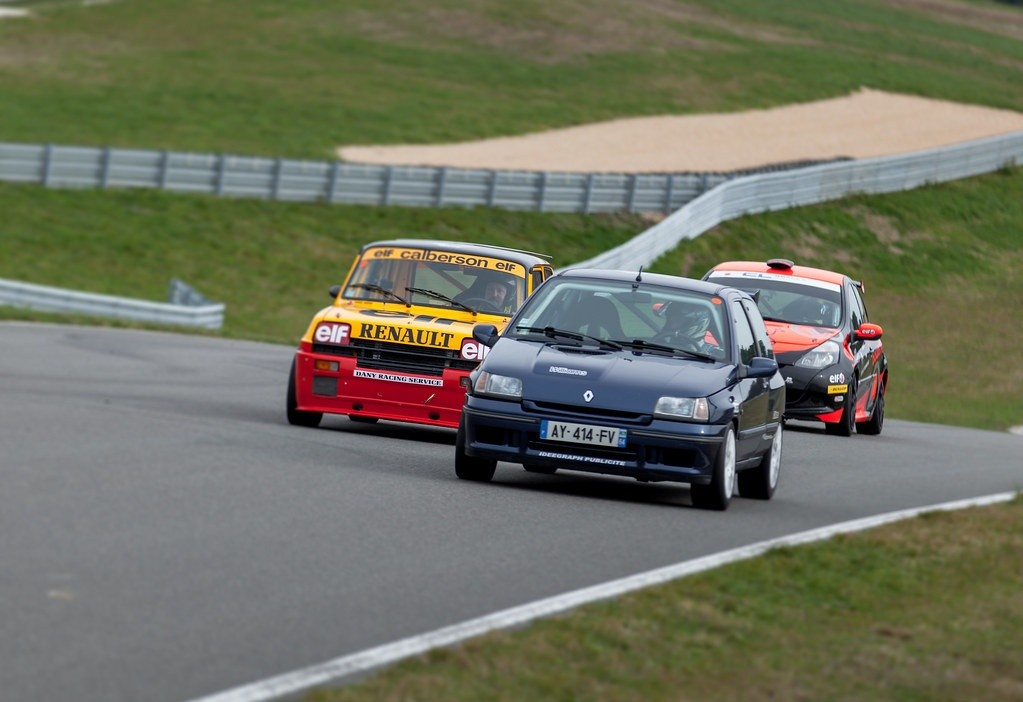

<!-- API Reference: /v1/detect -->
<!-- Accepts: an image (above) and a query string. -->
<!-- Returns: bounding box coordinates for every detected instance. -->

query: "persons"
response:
[657,300,725,360]
[464,274,517,312]
[800,295,828,326]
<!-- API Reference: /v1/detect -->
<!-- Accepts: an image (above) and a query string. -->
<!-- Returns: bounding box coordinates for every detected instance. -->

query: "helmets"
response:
[802,296,827,320]
[481,271,517,306]
[665,302,711,337]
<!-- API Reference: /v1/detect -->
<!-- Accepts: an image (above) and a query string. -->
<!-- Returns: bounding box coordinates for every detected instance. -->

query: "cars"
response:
[697,257,891,435]
[456,264,786,510]
[285,233,560,447]
